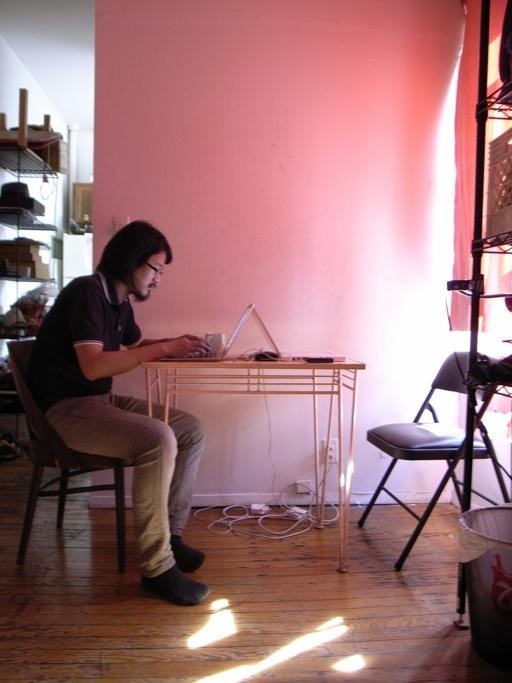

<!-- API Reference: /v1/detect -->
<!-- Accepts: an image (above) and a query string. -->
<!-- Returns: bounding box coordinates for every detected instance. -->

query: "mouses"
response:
[254,349,277,363]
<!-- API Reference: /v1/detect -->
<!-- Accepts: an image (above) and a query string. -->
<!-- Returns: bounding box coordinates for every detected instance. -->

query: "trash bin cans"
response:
[458,506,512,672]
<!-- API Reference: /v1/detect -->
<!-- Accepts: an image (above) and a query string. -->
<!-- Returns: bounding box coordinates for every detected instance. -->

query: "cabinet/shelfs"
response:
[1,142,71,456]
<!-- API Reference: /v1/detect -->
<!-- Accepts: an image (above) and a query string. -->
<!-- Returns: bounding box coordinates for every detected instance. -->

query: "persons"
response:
[27,219,213,608]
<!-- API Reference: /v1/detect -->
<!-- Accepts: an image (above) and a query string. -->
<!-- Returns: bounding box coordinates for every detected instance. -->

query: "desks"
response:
[139,356,366,577]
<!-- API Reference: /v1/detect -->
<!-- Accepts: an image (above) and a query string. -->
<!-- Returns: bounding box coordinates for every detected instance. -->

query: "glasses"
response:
[146,261,165,278]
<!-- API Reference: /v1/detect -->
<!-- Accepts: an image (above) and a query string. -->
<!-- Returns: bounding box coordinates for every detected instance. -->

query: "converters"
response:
[250,503,266,515]
[297,480,312,494]
[288,507,308,520]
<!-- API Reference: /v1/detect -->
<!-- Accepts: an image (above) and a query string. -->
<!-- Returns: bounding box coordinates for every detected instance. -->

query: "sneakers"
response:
[171,536,207,573]
[142,563,213,608]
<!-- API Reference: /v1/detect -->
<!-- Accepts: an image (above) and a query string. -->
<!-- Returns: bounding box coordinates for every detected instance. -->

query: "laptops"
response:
[249,305,347,363]
[140,302,256,361]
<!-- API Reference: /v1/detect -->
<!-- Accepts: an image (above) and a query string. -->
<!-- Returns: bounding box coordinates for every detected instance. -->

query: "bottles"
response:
[83,214,89,231]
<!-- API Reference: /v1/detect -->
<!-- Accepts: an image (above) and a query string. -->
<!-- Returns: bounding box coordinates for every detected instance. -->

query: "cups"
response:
[204,332,226,353]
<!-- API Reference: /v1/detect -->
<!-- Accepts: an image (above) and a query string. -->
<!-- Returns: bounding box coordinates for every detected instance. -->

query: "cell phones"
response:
[302,356,335,362]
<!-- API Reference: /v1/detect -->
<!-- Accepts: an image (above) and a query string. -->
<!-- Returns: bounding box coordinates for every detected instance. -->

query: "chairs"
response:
[1,339,129,576]
[356,351,510,573]
